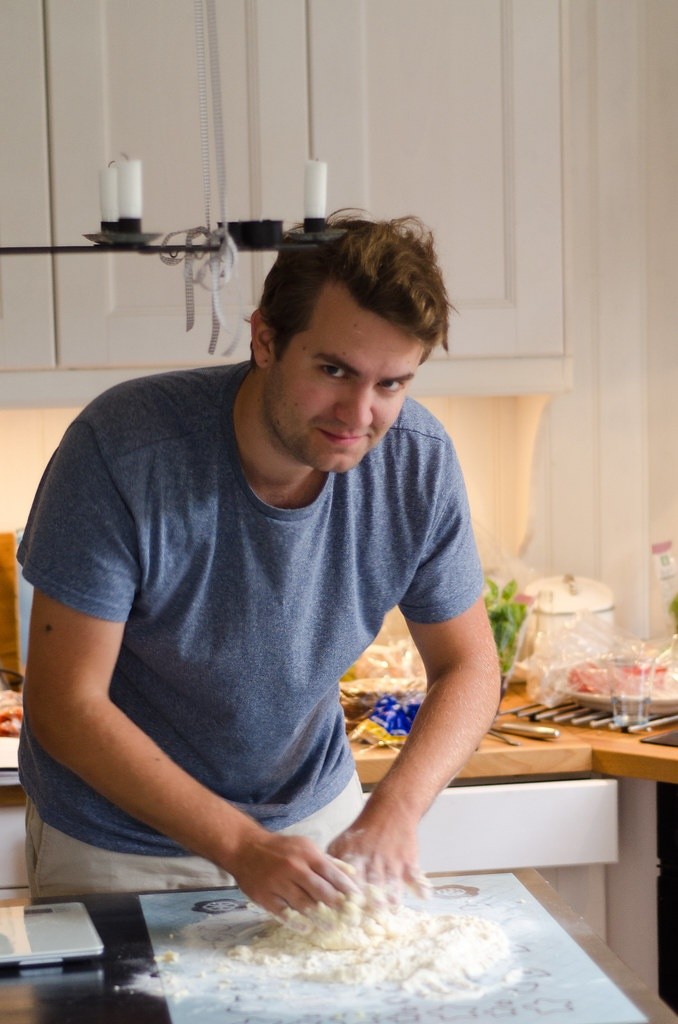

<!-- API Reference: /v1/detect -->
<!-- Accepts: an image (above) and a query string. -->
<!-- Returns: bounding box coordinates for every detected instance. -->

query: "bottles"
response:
[527,573,614,642]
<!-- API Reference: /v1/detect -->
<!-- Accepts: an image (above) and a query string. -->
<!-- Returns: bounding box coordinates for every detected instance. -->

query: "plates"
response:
[567,684,678,716]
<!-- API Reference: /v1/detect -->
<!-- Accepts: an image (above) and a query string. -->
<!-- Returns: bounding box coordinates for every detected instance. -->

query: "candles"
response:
[98,158,120,221]
[305,158,326,216]
[116,152,142,220]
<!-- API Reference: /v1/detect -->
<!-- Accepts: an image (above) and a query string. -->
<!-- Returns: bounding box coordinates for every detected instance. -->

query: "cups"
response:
[607,658,654,729]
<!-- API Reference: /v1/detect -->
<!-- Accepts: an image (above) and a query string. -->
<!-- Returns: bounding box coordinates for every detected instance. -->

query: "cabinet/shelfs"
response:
[0,0,576,412]
[1,772,662,1001]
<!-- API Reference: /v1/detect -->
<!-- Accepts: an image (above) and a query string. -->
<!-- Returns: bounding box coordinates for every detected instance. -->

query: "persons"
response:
[17,213,502,932]
